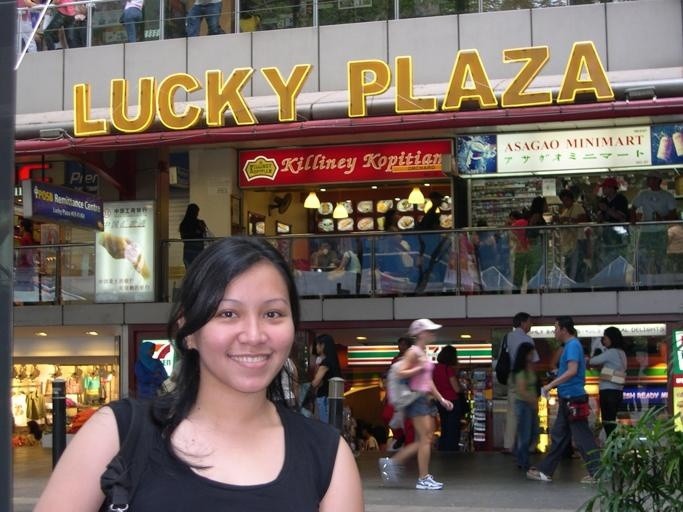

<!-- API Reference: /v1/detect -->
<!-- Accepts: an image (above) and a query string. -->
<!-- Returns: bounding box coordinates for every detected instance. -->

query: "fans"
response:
[268,193,292,216]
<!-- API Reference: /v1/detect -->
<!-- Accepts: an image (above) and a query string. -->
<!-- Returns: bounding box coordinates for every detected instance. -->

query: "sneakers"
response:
[415,474,444,490]
[378,457,402,487]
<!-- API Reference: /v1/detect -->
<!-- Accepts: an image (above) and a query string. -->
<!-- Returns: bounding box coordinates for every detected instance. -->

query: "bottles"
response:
[657,137,671,160]
[671,131,683,158]
[465,142,484,172]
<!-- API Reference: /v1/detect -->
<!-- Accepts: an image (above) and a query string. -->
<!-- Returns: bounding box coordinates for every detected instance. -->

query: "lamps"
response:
[303,189,321,209]
[332,202,349,219]
[408,184,425,204]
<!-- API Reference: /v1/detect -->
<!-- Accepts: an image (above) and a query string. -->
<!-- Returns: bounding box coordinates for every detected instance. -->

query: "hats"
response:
[407,318,443,336]
[644,171,661,179]
[604,178,619,189]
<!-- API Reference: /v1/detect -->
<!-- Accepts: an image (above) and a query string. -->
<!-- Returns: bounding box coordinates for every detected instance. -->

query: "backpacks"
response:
[386,358,421,410]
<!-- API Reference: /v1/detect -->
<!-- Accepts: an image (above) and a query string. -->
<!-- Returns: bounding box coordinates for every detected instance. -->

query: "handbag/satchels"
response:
[564,394,591,419]
[601,367,626,385]
[495,351,511,384]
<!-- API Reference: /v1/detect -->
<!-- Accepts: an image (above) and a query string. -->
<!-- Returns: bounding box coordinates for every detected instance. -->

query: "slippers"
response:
[526,469,553,483]
[581,475,610,484]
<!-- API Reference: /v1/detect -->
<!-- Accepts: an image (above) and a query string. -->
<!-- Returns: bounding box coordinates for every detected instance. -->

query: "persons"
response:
[179,204,208,270]
[14,1,263,54]
[360,426,378,451]
[33,234,365,512]
[135,342,168,396]
[379,318,454,491]
[276,334,341,423]
[334,242,362,273]
[419,190,452,231]
[311,241,338,268]
[499,312,628,483]
[381,338,413,450]
[474,174,679,294]
[384,209,400,230]
[20,219,39,266]
[433,346,467,452]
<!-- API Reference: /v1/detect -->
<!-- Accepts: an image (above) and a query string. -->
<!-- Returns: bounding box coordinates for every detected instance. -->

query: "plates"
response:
[397,216,414,229]
[397,198,413,212]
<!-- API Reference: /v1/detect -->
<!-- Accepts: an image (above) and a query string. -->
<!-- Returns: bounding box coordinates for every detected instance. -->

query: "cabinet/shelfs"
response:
[470,184,542,228]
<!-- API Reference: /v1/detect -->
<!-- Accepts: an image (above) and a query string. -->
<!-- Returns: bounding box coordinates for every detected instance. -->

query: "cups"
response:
[377,217,385,230]
